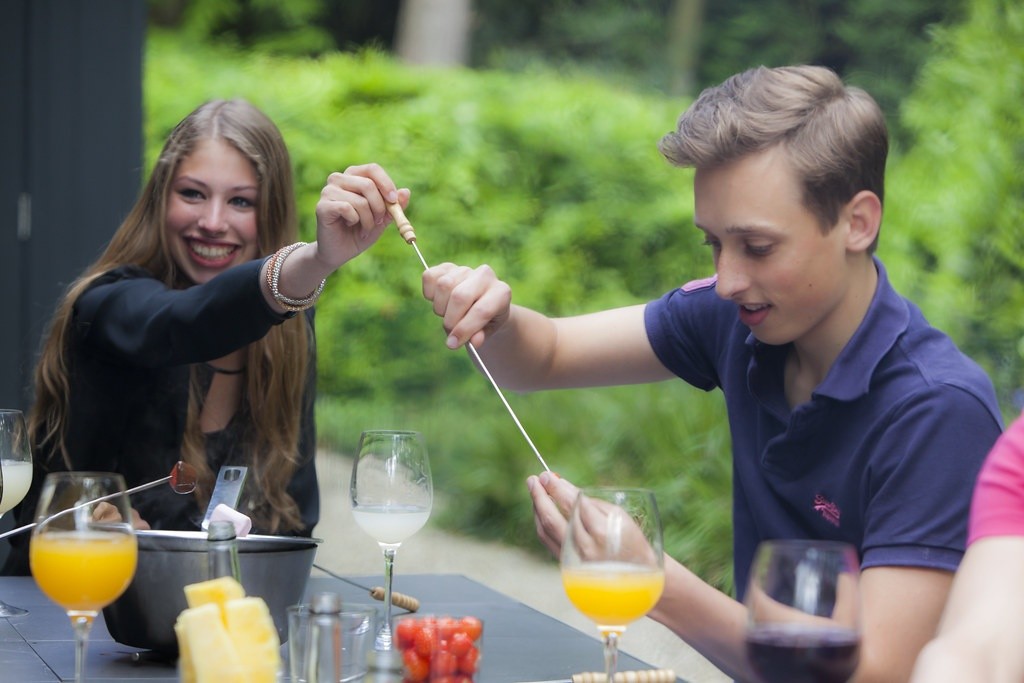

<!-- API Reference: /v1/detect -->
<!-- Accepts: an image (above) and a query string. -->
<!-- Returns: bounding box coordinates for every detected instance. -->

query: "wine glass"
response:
[0,409,38,616]
[349,430,434,660]
[27,471,138,682]
[560,486,669,682]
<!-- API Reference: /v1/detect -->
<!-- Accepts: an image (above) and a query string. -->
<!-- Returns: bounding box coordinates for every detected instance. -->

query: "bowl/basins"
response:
[94,521,325,652]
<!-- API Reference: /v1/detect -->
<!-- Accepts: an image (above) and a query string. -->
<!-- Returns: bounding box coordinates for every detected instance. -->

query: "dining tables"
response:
[1,567,692,682]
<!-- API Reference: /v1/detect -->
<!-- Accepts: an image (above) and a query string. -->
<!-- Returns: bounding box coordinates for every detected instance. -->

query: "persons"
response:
[423,61,1008,683]
[902,409,1024,681]
[1,99,414,581]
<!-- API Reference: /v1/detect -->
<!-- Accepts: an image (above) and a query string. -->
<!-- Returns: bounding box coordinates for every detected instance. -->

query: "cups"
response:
[741,539,863,683]
[289,601,372,683]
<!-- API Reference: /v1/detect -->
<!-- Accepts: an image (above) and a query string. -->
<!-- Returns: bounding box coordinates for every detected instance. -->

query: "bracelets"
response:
[268,247,319,312]
[272,242,328,306]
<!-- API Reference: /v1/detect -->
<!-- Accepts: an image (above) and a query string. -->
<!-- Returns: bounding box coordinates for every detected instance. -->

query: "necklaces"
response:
[205,361,247,375]
